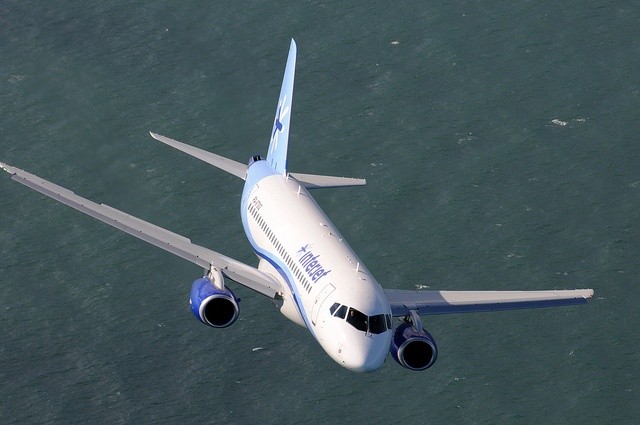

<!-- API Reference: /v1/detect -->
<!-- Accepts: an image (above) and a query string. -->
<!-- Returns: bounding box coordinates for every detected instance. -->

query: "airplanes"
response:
[0,39,595,374]
